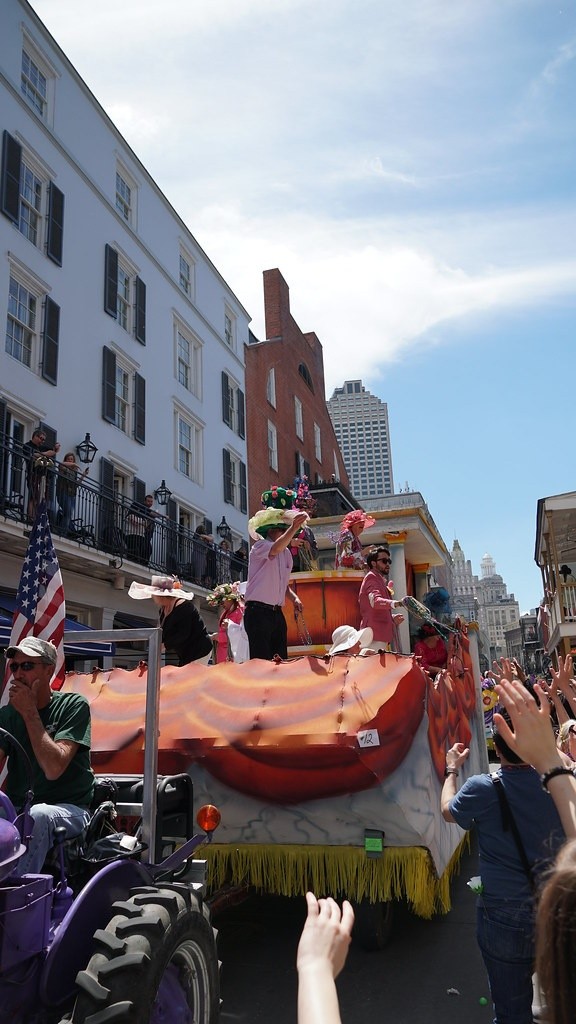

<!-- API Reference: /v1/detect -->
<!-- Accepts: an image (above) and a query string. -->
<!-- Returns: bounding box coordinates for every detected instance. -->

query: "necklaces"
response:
[300,546,318,571]
[403,595,460,642]
[294,609,313,646]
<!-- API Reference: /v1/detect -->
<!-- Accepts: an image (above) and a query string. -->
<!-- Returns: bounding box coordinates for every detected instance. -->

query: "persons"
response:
[558,565,576,622]
[359,548,404,650]
[209,597,245,662]
[243,509,307,660]
[192,526,212,582]
[0,636,96,879]
[140,495,161,565]
[288,525,319,573]
[124,502,147,563]
[217,541,232,583]
[24,430,57,524]
[231,546,247,582]
[295,680,576,1024]
[415,624,448,675]
[481,654,576,764]
[329,626,372,656]
[208,534,217,585]
[150,575,214,666]
[335,511,378,569]
[57,453,90,536]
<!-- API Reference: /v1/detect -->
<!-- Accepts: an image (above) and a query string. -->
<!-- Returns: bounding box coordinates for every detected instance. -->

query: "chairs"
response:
[0,489,25,522]
[57,510,94,545]
[106,525,140,558]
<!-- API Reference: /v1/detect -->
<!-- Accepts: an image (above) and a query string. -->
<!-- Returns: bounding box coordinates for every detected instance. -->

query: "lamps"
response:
[113,577,125,590]
[155,480,172,505]
[192,599,200,613]
[76,433,99,464]
[217,515,232,537]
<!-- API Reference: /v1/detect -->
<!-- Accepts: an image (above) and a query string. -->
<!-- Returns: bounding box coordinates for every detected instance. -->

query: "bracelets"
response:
[445,767,460,776]
[539,766,576,793]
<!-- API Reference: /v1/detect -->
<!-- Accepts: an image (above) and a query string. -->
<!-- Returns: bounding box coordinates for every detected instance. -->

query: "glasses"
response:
[375,558,392,565]
[35,434,45,442]
[9,662,52,673]
[568,724,576,736]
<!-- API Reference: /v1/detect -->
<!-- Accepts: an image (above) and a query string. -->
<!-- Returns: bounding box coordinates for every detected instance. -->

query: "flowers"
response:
[288,474,318,515]
[206,580,246,606]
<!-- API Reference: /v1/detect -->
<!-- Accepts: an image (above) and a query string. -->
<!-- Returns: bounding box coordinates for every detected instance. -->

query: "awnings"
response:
[0,594,115,658]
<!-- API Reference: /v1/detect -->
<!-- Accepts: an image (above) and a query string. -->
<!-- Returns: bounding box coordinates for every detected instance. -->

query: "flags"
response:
[0,508,66,798]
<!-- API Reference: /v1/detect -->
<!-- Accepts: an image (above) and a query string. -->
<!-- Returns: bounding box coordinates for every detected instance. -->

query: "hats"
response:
[409,622,450,641]
[247,486,308,540]
[5,635,58,666]
[205,584,240,605]
[324,625,374,655]
[126,574,194,601]
[558,564,572,575]
[340,509,375,529]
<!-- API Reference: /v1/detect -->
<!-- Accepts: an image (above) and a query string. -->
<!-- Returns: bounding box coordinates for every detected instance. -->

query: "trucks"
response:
[59,570,489,952]
